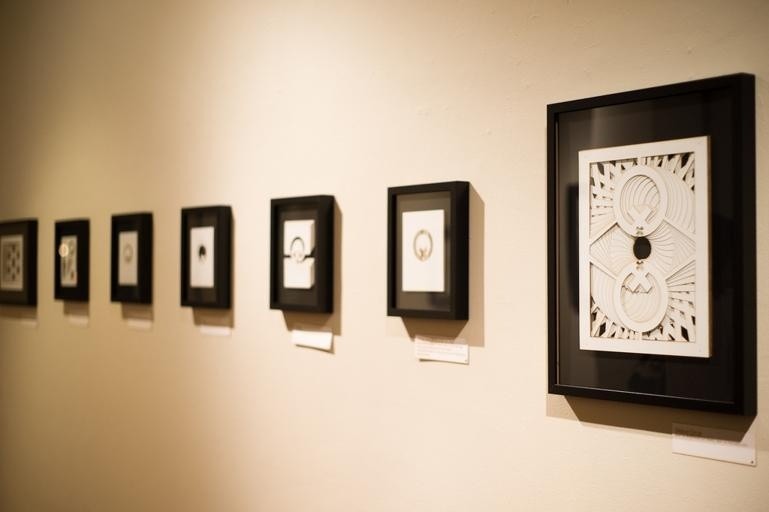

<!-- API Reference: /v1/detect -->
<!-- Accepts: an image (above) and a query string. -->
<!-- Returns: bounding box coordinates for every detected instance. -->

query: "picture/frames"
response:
[52,217,90,304]
[179,205,233,310]
[542,74,756,416]
[267,195,338,315]
[1,217,38,309]
[386,180,472,320]
[109,212,154,305]
